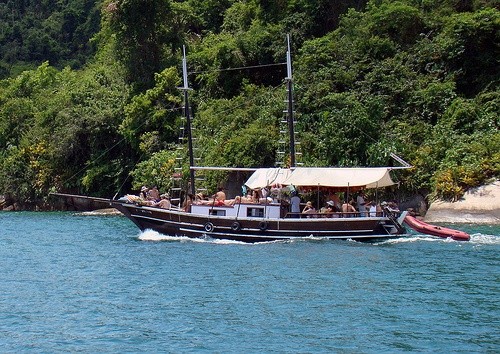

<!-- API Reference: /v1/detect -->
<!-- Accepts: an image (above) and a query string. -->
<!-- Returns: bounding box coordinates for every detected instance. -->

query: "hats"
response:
[327,200,335,207]
[164,193,170,199]
[160,193,164,197]
[140,186,148,191]
[307,201,312,207]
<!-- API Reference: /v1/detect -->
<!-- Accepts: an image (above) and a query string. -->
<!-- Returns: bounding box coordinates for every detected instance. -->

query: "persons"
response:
[139,185,171,210]
[288,190,400,218]
[178,185,274,213]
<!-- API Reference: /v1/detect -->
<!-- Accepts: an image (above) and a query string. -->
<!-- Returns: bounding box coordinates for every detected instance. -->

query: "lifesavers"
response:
[258,221,268,231]
[231,222,240,232]
[204,222,214,233]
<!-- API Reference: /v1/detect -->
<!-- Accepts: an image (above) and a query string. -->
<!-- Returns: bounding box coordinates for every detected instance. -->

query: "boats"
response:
[48,94,185,211]
[403,214,471,241]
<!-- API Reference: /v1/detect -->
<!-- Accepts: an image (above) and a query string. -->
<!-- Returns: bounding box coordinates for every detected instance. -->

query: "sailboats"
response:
[108,34,411,242]
